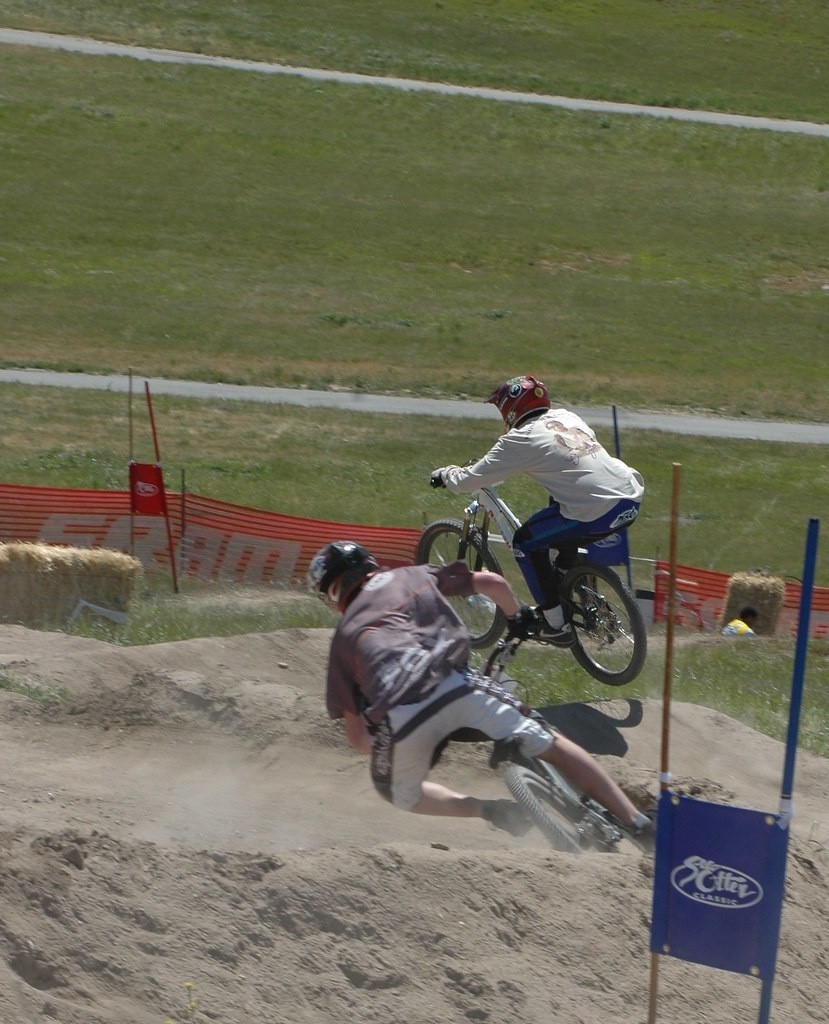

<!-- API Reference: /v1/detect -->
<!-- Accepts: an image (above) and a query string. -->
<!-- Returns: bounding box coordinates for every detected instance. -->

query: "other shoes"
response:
[492,800,529,836]
[634,812,657,840]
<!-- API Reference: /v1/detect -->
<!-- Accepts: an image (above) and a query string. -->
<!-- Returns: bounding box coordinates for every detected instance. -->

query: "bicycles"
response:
[474,610,657,856]
[410,459,649,686]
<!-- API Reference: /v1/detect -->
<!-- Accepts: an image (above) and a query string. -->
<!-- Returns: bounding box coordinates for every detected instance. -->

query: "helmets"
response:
[484,375,551,430]
[307,541,378,614]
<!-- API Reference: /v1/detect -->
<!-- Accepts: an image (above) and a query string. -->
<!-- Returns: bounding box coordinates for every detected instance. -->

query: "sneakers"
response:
[526,617,578,648]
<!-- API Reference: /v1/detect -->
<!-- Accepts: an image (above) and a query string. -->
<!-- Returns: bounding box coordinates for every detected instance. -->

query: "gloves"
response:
[431,467,447,489]
[506,606,538,641]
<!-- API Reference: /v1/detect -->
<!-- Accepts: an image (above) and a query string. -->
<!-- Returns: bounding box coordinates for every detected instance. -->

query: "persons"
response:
[719,607,759,639]
[305,538,655,840]
[428,375,646,647]
